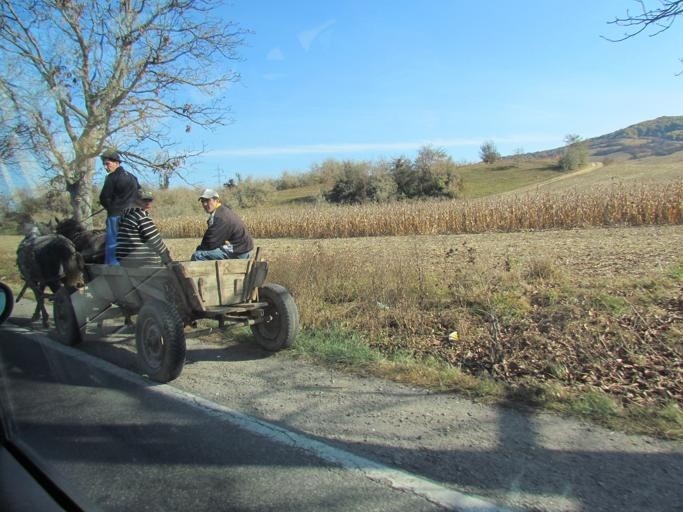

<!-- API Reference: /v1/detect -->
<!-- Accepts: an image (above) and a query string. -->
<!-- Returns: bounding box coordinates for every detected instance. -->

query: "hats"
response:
[101,150,126,162]
[137,189,154,199]
[198,189,219,201]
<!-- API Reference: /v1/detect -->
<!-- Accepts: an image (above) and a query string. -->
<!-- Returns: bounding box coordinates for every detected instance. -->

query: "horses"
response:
[55,216,133,328]
[16,219,85,327]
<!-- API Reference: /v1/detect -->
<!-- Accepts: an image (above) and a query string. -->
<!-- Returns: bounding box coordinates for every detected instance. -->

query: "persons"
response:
[114,189,171,268]
[99,149,141,265]
[191,188,254,261]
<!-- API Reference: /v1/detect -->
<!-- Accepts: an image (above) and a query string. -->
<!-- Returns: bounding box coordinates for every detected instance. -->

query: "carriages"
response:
[16,214,300,382]
